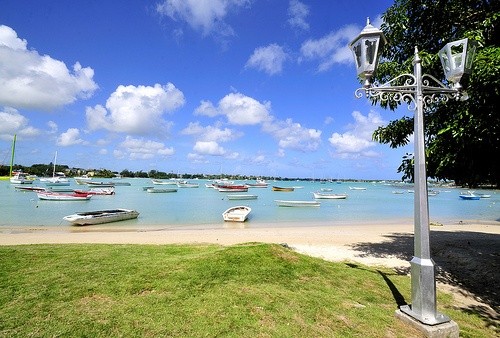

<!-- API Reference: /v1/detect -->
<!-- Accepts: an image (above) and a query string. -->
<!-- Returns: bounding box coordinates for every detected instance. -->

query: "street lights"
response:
[348,17,476,338]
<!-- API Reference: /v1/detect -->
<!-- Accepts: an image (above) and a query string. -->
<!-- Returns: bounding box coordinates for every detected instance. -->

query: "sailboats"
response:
[310,175,341,184]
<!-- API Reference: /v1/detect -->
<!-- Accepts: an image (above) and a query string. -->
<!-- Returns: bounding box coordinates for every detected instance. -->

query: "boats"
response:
[274,200,320,208]
[206,164,271,192]
[392,185,491,200]
[143,178,199,193]
[226,195,258,200]
[273,185,304,192]
[10,151,130,201]
[350,186,366,190]
[222,206,251,222]
[312,188,346,199]
[63,208,140,226]
